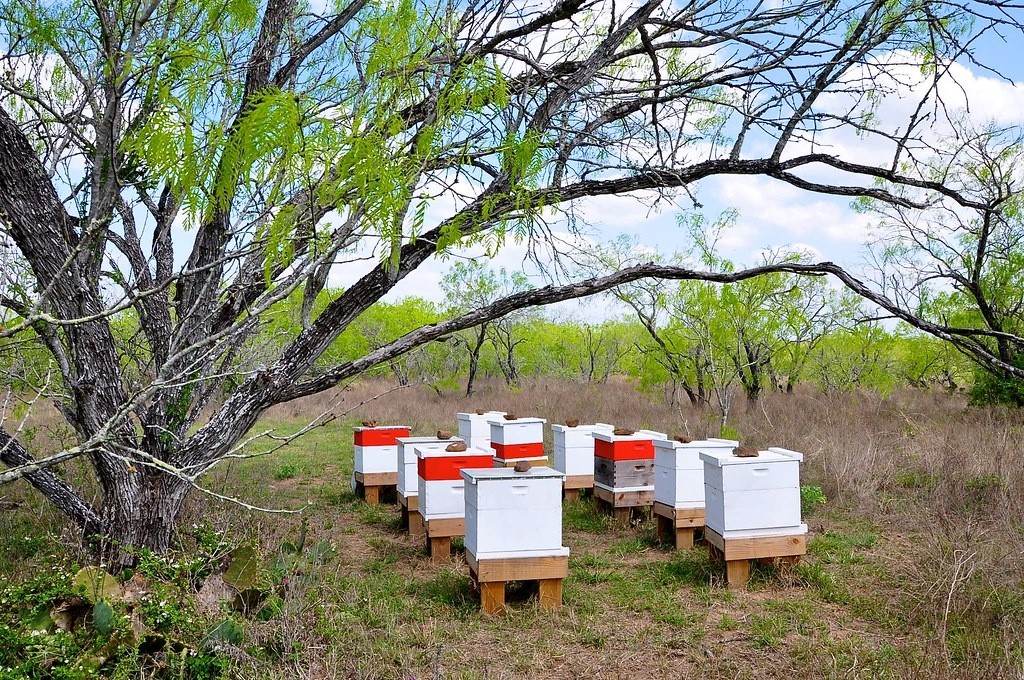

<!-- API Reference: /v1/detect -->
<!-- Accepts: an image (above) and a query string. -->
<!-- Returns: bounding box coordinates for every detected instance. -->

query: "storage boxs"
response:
[353,411,810,564]
